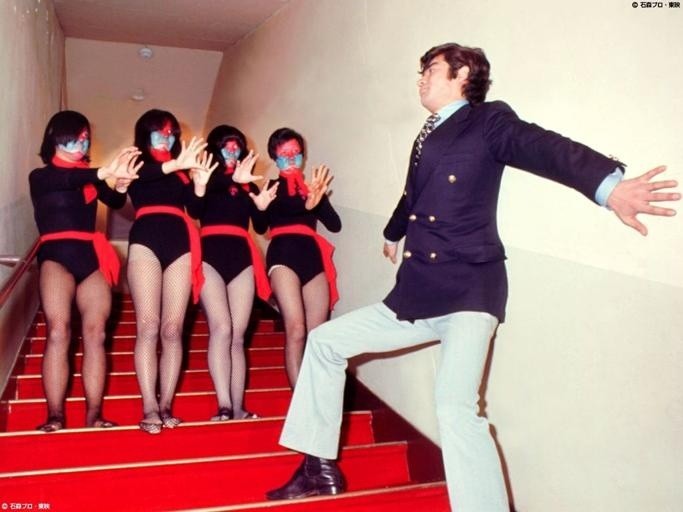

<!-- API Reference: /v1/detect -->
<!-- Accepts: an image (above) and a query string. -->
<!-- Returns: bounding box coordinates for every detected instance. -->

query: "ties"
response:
[412,113,439,164]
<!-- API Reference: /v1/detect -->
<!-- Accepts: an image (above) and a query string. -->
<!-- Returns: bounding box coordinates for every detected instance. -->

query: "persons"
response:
[262,128,341,390]
[266,42,679,511]
[187,123,278,423]
[28,109,143,431]
[124,106,208,436]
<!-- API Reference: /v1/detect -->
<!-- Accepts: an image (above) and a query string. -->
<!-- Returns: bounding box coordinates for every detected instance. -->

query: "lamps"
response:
[130,88,145,101]
[138,44,152,59]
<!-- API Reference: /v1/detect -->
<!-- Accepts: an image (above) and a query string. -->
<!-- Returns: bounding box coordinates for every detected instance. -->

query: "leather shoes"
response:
[263,455,344,500]
